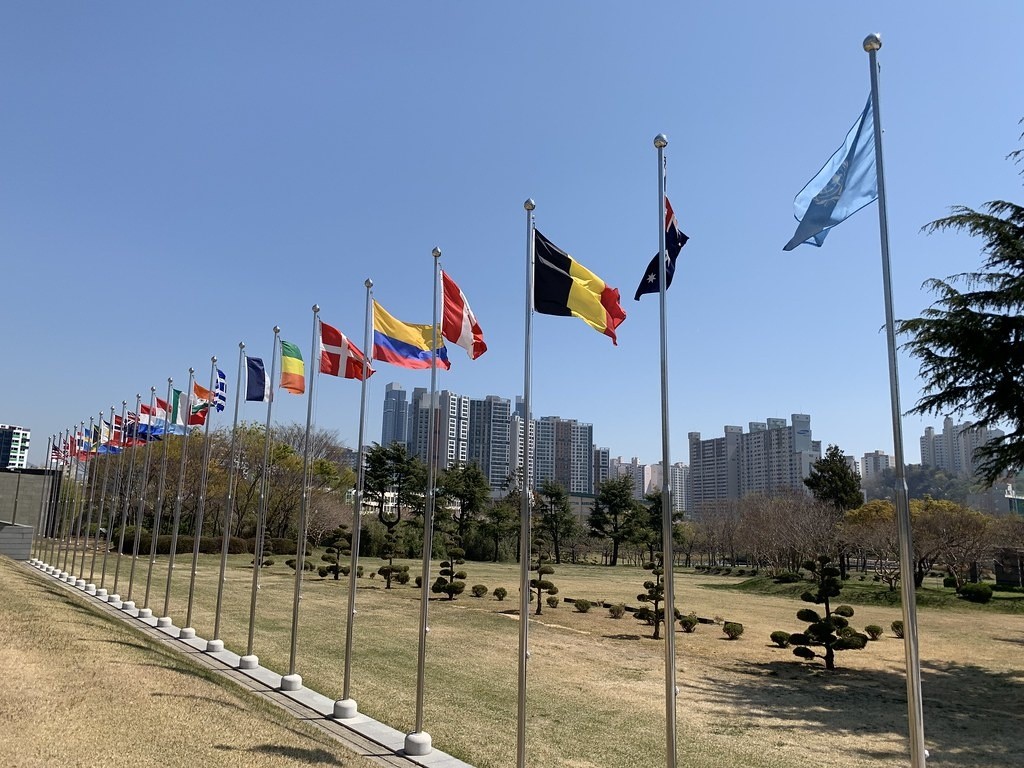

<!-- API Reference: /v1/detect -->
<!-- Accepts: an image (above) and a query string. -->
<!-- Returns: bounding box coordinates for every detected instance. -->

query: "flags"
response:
[187,381,215,425]
[316,320,377,381]
[531,228,627,346]
[278,340,306,394]
[214,368,226,412]
[244,356,274,403]
[633,156,689,300]
[371,299,452,371]
[440,268,489,361]
[47,388,193,466]
[781,65,882,252]
